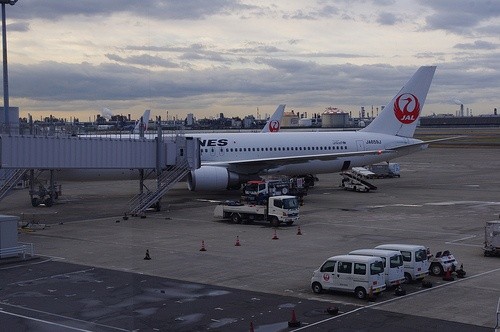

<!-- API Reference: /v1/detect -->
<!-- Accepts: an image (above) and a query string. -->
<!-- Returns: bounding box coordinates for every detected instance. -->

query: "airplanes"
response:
[76,65,438,193]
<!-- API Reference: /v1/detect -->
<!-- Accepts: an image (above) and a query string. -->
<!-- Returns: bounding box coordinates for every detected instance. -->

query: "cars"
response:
[389,162,401,178]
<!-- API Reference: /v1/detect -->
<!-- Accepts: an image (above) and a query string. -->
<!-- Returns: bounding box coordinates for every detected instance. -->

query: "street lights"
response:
[0,0,20,134]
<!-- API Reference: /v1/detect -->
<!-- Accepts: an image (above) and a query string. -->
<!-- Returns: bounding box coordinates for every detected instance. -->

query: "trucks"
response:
[213,196,304,228]
[482,220,500,257]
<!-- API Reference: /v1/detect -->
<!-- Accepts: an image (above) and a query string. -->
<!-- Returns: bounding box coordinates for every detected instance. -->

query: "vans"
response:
[374,243,430,284]
[311,255,387,300]
[343,248,406,287]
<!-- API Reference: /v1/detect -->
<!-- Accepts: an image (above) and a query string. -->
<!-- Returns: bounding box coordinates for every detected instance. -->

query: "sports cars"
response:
[429,250,458,277]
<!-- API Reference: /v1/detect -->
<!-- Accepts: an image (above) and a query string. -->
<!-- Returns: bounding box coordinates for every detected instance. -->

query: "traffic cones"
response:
[296,226,303,235]
[234,235,241,246]
[271,229,279,240]
[291,309,297,320]
[249,320,255,332]
[199,240,208,251]
[143,248,152,260]
[445,267,451,279]
